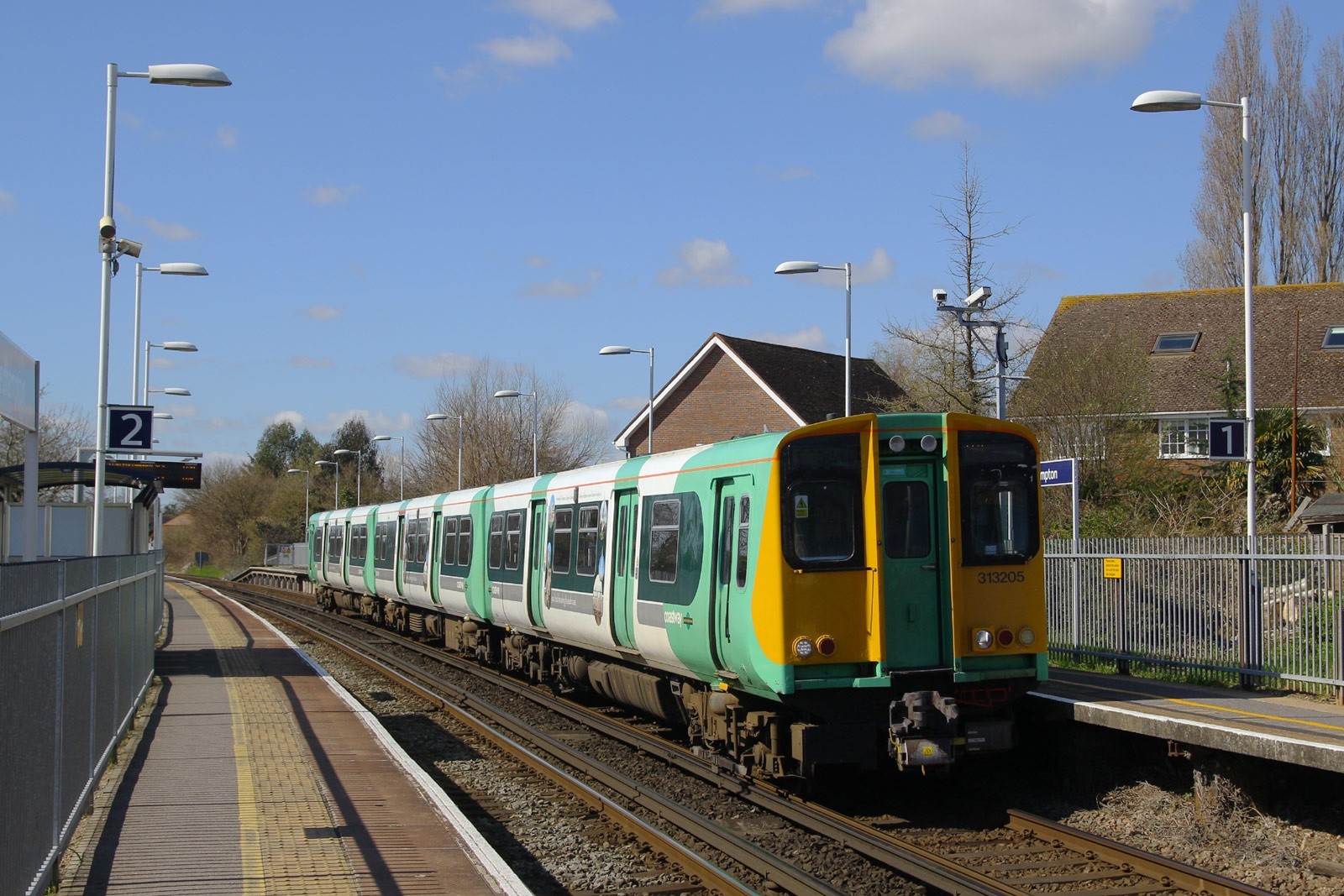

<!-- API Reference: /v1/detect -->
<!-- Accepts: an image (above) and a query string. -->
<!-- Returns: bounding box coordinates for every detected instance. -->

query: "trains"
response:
[305,408,1051,807]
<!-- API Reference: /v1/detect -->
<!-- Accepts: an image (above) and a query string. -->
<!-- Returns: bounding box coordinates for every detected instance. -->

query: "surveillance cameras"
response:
[117,239,143,258]
[933,288,947,302]
[965,287,993,306]
[99,216,116,238]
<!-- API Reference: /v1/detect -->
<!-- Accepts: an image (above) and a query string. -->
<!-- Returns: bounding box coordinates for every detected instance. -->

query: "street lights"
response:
[130,262,210,406]
[773,259,851,419]
[314,460,338,511]
[425,414,463,490]
[372,435,404,501]
[89,63,233,555]
[494,390,539,478]
[287,469,310,542]
[598,345,654,454]
[1130,87,1258,692]
[143,339,199,406]
[333,449,360,507]
